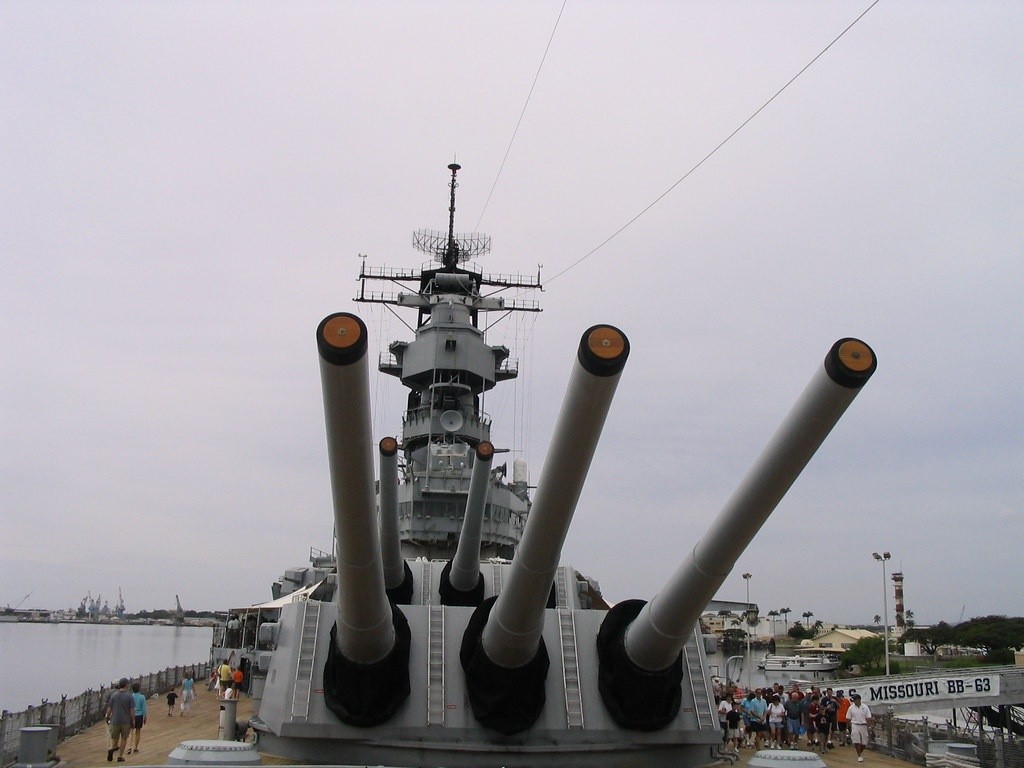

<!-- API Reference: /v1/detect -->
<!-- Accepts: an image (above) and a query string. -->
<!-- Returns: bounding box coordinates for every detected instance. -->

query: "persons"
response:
[718,683,850,753]
[166,687,178,716]
[180,672,196,716]
[232,666,243,701]
[218,659,231,695]
[845,694,873,762]
[228,614,275,651]
[127,683,148,753]
[106,678,136,763]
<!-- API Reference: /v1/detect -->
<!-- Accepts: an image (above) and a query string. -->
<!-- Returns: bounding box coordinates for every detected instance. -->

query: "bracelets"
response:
[869,727,871,728]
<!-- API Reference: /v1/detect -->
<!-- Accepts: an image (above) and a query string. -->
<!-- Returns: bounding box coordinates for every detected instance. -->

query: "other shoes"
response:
[815,740,820,746]
[764,740,770,747]
[839,742,845,746]
[113,746,119,751]
[820,749,825,754]
[770,742,775,749]
[827,743,835,749]
[776,745,781,750]
[126,749,131,754]
[735,748,739,752]
[107,749,113,761]
[858,757,863,762]
[790,743,799,750]
[807,742,810,746]
[117,756,125,762]
[134,749,139,752]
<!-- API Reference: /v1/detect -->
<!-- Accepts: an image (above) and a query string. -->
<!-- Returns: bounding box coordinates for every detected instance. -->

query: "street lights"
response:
[872,551,893,677]
[742,572,753,691]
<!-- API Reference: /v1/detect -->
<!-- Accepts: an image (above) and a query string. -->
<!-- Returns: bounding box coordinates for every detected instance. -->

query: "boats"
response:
[758,650,840,674]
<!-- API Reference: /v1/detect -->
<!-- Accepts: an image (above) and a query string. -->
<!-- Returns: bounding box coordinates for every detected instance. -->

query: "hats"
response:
[806,689,812,694]
[113,679,119,686]
[852,694,860,699]
